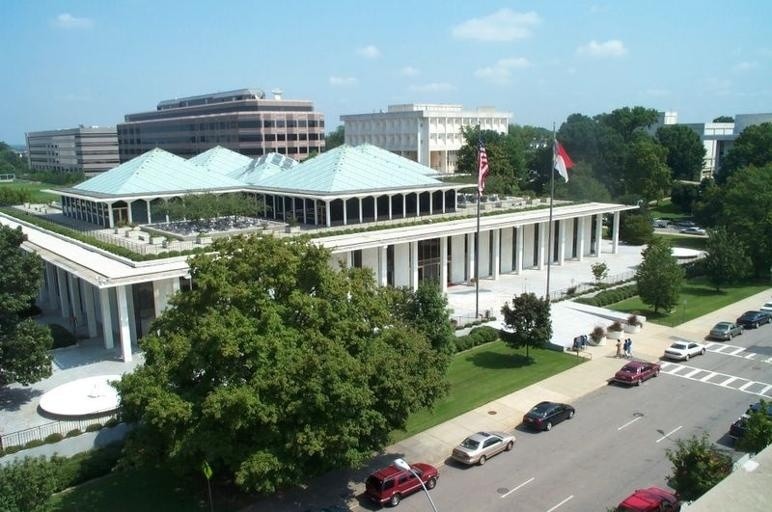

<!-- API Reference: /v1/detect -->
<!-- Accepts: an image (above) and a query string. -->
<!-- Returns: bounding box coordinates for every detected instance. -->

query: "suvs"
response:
[366,462,440,506]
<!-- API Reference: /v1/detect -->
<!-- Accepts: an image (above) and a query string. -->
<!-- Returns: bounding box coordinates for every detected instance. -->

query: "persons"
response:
[616,338,632,359]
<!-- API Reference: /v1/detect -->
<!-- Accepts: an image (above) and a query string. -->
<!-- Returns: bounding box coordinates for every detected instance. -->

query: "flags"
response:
[554,139,576,183]
[479,137,490,192]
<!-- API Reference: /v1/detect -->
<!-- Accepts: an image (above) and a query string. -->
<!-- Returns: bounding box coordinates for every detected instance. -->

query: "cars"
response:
[614,361,662,388]
[678,219,707,236]
[737,311,770,328]
[452,430,516,466]
[613,485,682,512]
[710,322,743,340]
[760,302,772,316]
[650,216,671,227]
[728,401,771,447]
[664,341,706,361]
[522,401,576,431]
